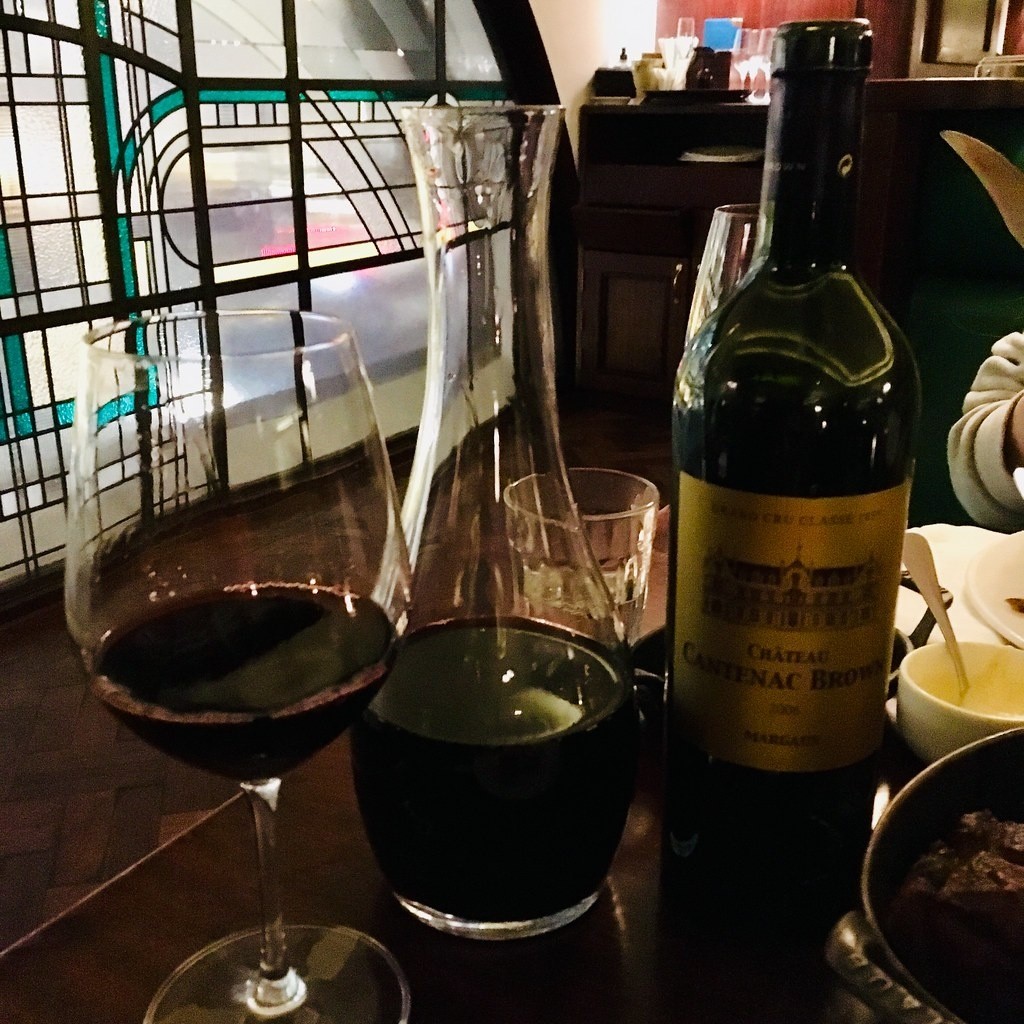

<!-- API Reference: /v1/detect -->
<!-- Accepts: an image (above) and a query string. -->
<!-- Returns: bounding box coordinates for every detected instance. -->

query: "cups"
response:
[498,465,657,657]
[653,68,676,92]
[630,58,663,93]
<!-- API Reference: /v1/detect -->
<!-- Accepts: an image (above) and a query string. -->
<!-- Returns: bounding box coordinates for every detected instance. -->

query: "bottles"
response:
[618,48,630,70]
[656,17,923,934]
[342,106,633,937]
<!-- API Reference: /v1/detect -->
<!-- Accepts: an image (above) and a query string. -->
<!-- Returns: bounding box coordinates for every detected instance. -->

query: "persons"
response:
[947,330,1024,535]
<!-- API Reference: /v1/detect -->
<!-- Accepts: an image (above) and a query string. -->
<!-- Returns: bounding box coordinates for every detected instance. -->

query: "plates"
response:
[590,96,632,105]
[966,529,1024,649]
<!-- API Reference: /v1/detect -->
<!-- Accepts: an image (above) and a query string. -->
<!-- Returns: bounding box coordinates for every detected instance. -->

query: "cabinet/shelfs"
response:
[577,249,702,409]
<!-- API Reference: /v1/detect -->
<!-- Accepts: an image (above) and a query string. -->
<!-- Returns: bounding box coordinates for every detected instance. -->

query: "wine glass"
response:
[665,15,776,89]
[682,199,762,352]
[65,308,409,1023]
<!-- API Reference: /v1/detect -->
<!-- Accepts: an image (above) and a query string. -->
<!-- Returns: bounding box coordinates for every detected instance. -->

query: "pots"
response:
[828,730,1023,1024]
[591,70,634,98]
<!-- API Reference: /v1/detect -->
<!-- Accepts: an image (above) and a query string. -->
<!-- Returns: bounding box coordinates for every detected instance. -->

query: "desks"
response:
[0,502,1024,1023]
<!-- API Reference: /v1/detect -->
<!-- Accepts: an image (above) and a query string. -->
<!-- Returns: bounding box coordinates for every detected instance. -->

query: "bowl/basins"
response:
[899,639,1024,759]
